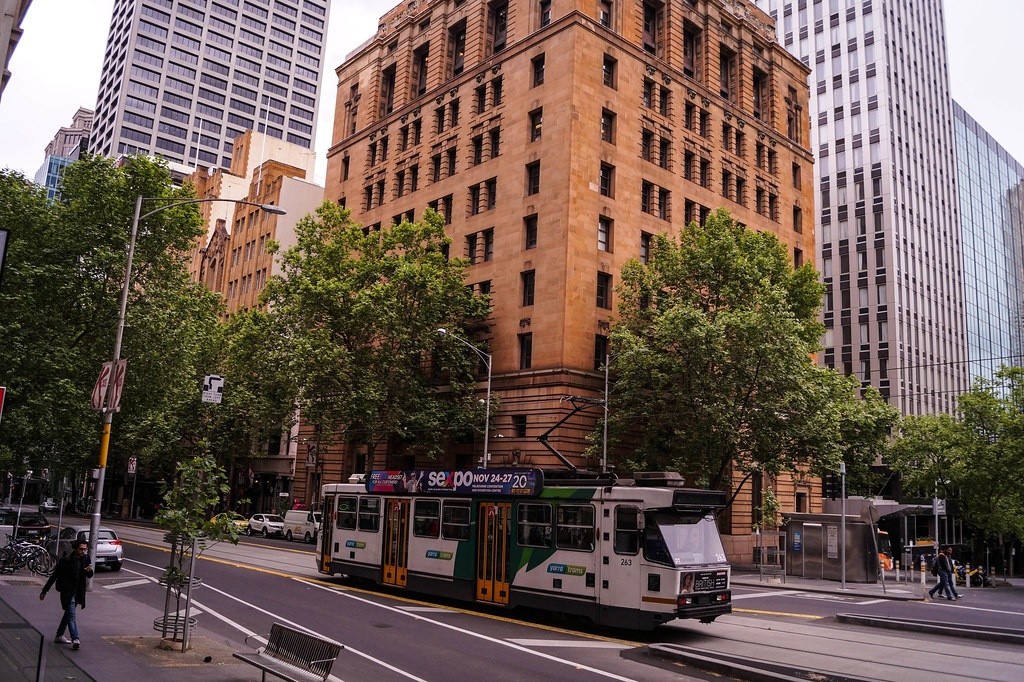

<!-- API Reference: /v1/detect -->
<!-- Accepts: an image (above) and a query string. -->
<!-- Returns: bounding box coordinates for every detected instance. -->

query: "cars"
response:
[210,507,247,538]
[0,505,51,540]
[248,510,284,541]
[47,524,123,568]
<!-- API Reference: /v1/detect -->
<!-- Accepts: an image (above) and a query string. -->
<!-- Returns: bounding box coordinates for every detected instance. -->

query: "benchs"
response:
[232,623,345,682]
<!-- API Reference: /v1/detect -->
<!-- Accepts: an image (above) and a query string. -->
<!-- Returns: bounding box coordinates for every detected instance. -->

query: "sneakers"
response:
[73,639,80,649]
[55,635,71,644]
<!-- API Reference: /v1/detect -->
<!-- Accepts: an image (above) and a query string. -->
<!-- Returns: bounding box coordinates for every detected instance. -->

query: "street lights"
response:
[436,328,493,468]
[85,189,287,591]
[603,348,650,476]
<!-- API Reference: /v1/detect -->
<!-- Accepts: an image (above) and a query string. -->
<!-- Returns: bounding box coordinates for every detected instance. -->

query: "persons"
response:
[681,573,693,594]
[39,468,50,505]
[39,540,94,648]
[927,547,965,600]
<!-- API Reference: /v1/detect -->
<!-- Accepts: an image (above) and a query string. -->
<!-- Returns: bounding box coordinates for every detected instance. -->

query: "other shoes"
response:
[938,594,947,598]
[948,598,956,601]
[928,591,935,599]
[956,595,964,599]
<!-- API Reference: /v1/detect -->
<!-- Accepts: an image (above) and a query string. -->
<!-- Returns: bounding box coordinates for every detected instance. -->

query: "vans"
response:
[284,506,321,543]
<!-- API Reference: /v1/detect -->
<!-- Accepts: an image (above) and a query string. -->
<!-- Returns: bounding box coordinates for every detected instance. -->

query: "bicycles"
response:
[1,534,57,578]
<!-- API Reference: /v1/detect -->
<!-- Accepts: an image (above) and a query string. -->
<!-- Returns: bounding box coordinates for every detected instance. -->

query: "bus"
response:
[311,465,739,636]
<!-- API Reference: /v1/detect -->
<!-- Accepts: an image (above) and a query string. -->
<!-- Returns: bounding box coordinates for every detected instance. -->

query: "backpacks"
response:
[55,555,88,592]
